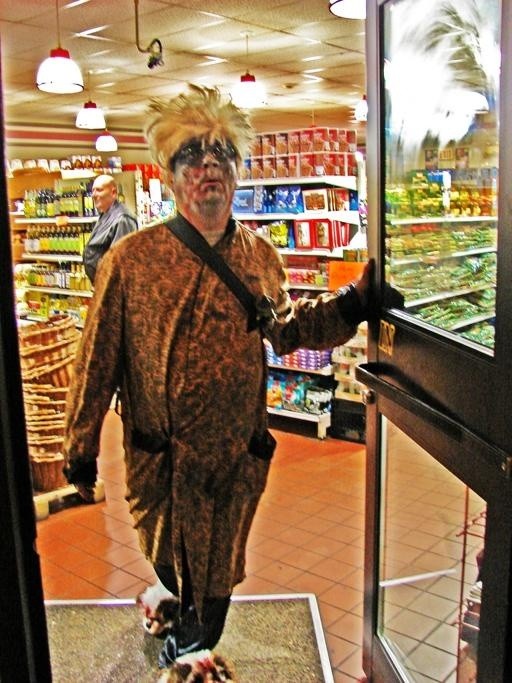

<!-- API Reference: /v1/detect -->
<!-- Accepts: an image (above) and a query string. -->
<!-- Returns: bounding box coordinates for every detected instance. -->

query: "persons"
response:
[59,81,406,682]
[79,174,142,289]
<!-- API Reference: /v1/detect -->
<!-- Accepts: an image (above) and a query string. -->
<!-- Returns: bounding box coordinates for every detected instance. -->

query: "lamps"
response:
[75,70,106,130]
[36,1,84,95]
[95,119,118,153]
[327,0,367,19]
[355,61,368,122]
[236,29,262,108]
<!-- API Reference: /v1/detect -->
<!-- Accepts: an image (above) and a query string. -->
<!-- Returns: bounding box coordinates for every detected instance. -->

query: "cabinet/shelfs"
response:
[6,172,174,521]
[232,172,497,437]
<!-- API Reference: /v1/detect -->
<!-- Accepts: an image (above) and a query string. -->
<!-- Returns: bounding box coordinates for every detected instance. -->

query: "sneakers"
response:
[139,580,181,636]
[155,649,234,683]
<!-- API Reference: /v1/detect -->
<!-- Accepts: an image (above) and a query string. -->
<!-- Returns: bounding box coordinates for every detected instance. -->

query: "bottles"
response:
[24,180,99,290]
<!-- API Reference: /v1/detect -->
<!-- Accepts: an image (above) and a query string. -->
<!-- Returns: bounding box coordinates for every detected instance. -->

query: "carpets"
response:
[42,593,335,683]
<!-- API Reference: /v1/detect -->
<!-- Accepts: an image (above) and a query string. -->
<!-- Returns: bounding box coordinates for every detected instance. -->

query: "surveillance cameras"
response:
[148,52,166,68]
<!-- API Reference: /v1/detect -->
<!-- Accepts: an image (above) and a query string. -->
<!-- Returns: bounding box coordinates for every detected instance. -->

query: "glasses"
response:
[175,139,237,165]
